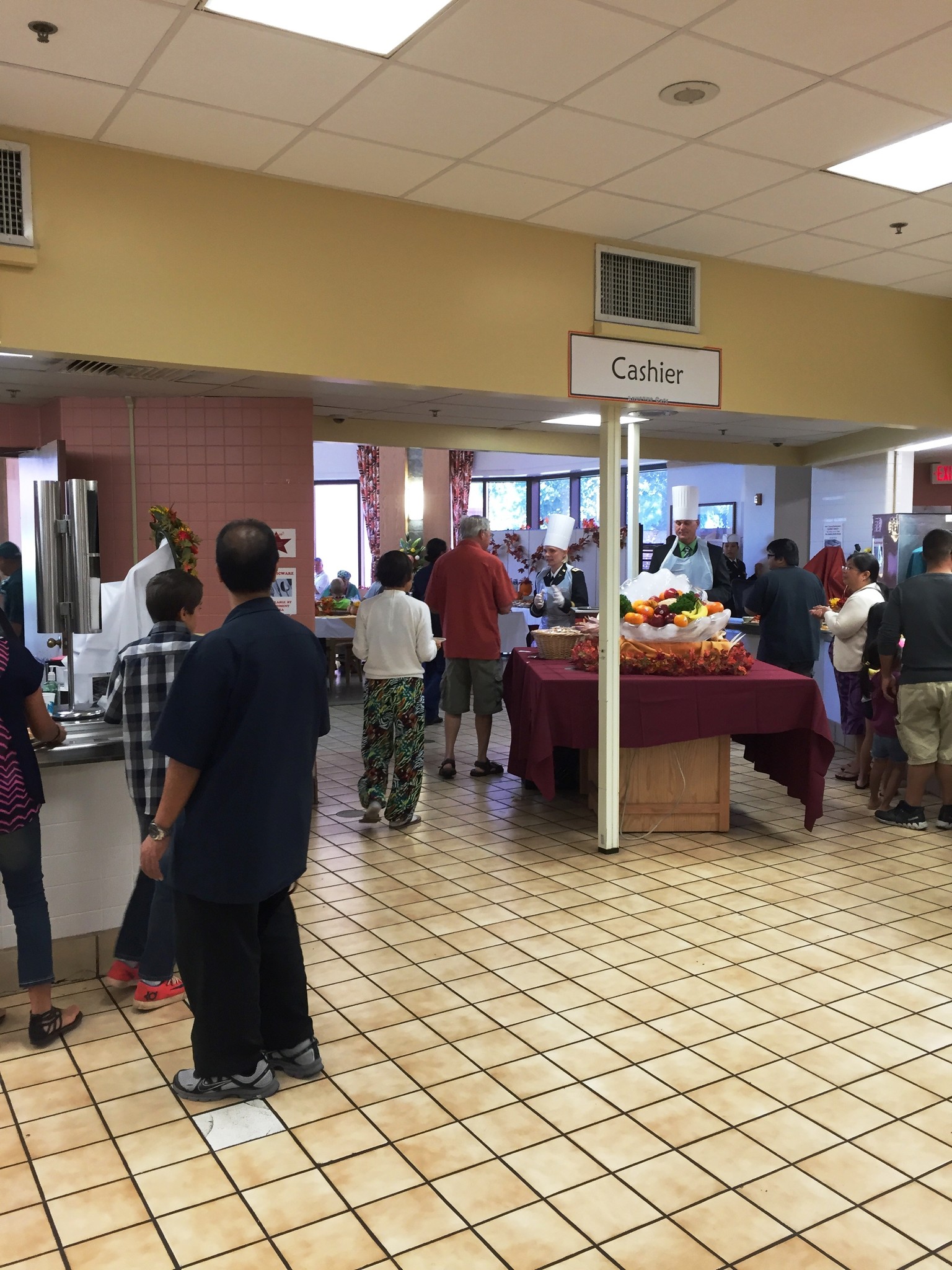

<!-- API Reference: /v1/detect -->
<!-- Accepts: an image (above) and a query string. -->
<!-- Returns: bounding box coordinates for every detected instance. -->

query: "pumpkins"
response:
[519,583,532,596]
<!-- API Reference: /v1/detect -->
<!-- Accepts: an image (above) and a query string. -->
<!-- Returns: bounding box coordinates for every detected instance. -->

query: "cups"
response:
[42,692,55,716]
[512,578,518,592]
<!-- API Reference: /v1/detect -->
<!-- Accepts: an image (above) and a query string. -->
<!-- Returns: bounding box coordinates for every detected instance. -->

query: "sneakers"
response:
[133,975,188,1010]
[105,960,141,989]
[935,806,952,829]
[172,1051,279,1100]
[262,1037,325,1080]
[875,799,928,830]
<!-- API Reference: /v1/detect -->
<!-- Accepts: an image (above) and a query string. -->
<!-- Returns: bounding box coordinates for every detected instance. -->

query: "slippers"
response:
[855,780,869,790]
[835,763,859,781]
[358,813,382,823]
[389,814,422,829]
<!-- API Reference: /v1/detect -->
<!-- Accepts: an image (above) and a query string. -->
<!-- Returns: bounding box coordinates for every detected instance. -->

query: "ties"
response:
[732,561,738,568]
[550,576,554,586]
[683,546,691,558]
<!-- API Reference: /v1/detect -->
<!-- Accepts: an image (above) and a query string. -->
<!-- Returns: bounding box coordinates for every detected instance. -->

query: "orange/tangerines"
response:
[703,602,724,616]
[674,614,688,627]
[625,590,683,625]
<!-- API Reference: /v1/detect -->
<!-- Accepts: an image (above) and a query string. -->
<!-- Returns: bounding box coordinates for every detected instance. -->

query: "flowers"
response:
[400,533,426,571]
[148,501,202,577]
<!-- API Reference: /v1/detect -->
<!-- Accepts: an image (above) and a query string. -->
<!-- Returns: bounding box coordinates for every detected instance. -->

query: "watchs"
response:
[149,817,175,841]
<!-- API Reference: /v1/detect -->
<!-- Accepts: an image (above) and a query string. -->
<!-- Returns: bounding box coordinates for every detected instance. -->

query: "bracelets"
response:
[49,723,61,741]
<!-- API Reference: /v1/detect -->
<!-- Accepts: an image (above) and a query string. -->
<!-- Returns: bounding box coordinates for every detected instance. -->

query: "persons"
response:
[809,552,886,781]
[743,538,827,679]
[0,541,25,646]
[647,486,732,602]
[529,513,589,647]
[424,516,518,777]
[314,538,449,726]
[0,637,83,1048]
[875,530,952,830]
[860,602,907,813]
[721,533,762,619]
[352,550,442,828]
[104,568,205,1011]
[140,519,330,1103]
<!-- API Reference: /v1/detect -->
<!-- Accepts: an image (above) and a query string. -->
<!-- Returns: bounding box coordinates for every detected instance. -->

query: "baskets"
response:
[530,626,584,660]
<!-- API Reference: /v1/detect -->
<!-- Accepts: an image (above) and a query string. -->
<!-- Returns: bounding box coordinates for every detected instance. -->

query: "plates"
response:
[820,622,830,631]
[432,637,447,642]
[741,621,760,626]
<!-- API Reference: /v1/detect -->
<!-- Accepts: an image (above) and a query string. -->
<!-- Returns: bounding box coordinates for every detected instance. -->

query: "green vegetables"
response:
[668,590,701,614]
[619,594,636,618]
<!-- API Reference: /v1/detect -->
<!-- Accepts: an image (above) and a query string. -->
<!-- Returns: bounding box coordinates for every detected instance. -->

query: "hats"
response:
[337,570,351,581]
[672,485,699,521]
[722,534,741,547]
[543,514,576,550]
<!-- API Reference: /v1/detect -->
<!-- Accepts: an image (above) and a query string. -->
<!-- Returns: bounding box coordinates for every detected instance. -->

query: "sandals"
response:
[29,1005,83,1049]
[470,757,504,777]
[438,758,457,775]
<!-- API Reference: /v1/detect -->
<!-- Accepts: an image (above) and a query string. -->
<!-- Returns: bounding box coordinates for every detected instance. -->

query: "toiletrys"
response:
[43,665,60,706]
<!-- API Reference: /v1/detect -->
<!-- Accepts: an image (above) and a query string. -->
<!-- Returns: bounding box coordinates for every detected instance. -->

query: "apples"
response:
[648,588,679,627]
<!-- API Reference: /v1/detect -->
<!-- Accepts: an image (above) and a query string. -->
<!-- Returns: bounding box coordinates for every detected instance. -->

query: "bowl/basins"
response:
[742,616,754,621]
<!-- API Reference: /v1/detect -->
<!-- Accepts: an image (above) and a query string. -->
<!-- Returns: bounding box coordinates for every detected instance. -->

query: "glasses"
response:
[767,554,775,559]
[841,565,860,573]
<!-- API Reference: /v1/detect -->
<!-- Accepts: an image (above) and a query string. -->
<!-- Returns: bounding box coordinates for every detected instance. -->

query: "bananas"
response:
[681,599,708,622]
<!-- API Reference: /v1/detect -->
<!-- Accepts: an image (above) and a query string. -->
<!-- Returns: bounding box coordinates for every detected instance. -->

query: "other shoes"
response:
[424,717,443,725]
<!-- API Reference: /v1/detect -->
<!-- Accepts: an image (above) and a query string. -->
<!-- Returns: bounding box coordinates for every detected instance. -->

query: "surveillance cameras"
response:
[770,439,786,447]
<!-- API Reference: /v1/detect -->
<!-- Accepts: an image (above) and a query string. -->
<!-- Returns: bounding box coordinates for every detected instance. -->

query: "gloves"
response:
[552,584,565,608]
[534,588,545,609]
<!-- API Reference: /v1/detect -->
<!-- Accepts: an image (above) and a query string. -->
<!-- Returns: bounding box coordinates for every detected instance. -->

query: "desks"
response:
[315,614,357,690]
[498,607,542,653]
[502,647,835,834]
